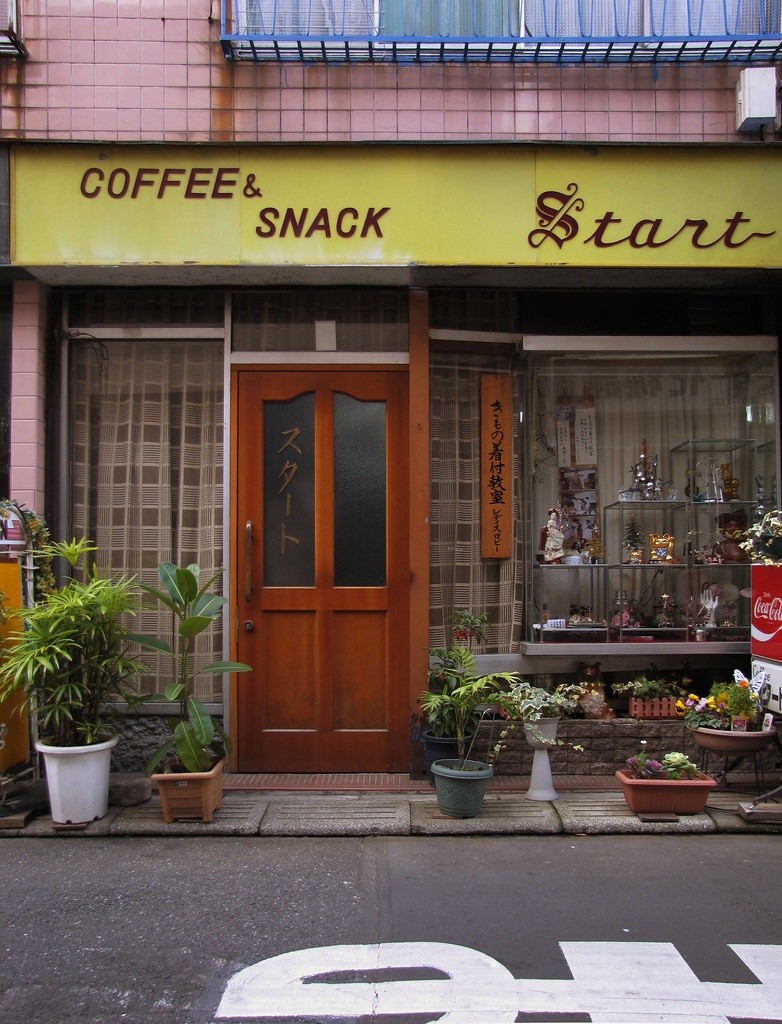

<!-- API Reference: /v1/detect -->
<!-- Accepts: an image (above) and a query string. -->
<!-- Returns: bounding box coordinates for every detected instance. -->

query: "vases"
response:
[760,533,782,559]
[692,726,777,750]
[628,696,687,720]
[615,770,717,813]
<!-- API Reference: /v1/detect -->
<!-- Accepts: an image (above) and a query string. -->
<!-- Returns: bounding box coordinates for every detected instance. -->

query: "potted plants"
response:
[0,536,153,823]
[113,561,253,823]
[488,681,606,802]
[417,610,523,817]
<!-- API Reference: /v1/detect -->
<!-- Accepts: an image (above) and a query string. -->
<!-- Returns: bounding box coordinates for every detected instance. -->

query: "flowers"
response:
[674,680,763,731]
[626,740,702,780]
[724,510,782,567]
[0,495,58,601]
[612,673,688,700]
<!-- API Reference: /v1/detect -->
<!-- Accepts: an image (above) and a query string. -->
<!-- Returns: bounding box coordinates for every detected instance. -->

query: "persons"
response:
[545,509,564,564]
[714,508,749,563]
[701,590,719,627]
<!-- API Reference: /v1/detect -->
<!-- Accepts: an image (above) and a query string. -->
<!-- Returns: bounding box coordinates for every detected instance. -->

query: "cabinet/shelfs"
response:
[533,562,610,643]
[603,500,689,643]
[670,439,757,642]
